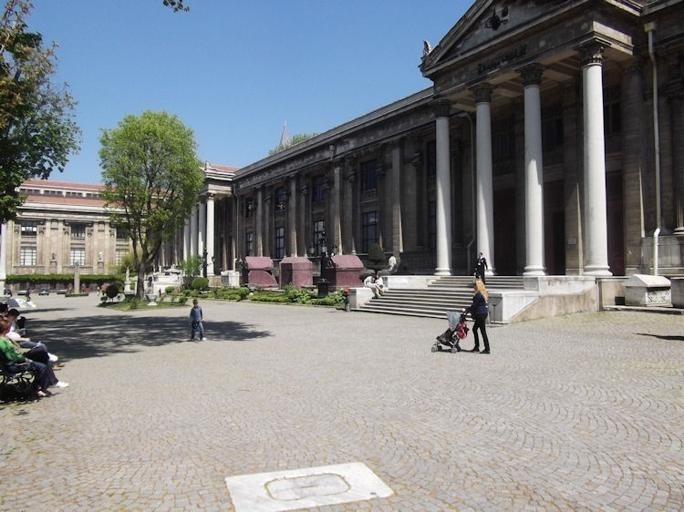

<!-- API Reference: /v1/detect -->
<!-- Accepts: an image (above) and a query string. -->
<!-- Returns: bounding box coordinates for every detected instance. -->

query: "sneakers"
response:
[37,390,49,397]
[471,347,479,352]
[480,350,490,354]
[48,353,58,362]
[51,381,70,388]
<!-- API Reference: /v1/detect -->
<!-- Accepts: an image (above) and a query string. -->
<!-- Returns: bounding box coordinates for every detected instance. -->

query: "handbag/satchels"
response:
[456,317,469,339]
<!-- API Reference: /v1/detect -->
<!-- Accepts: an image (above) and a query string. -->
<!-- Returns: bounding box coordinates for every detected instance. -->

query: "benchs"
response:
[0,361,37,406]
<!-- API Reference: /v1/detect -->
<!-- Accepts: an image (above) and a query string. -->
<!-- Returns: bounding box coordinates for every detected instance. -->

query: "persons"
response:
[190,300,206,341]
[364,254,397,297]
[475,252,488,285]
[464,279,490,354]
[0,287,69,396]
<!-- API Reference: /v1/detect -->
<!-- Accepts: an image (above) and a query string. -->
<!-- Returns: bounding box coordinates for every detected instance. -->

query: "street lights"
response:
[196,247,217,278]
[307,225,337,297]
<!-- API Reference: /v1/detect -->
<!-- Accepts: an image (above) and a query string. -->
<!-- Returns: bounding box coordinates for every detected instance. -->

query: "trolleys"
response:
[430,307,473,352]
